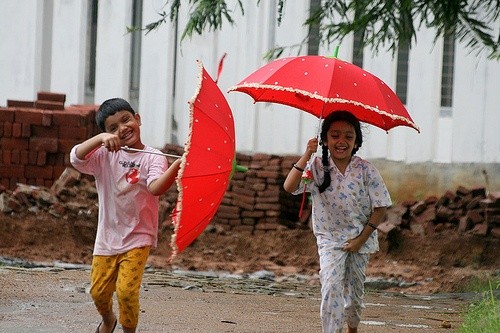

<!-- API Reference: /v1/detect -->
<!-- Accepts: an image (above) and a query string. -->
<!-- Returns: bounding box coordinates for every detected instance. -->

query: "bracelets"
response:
[366,221,379,231]
[292,160,305,173]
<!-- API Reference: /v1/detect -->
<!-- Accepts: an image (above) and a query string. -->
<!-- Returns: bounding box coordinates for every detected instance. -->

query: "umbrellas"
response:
[224,44,422,182]
[105,57,248,275]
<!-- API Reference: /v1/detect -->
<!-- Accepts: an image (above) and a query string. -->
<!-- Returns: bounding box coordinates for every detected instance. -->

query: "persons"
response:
[67,96,182,333]
[276,108,392,333]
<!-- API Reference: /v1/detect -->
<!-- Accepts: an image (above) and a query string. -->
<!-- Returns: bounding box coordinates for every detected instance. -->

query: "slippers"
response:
[95,316,118,333]
